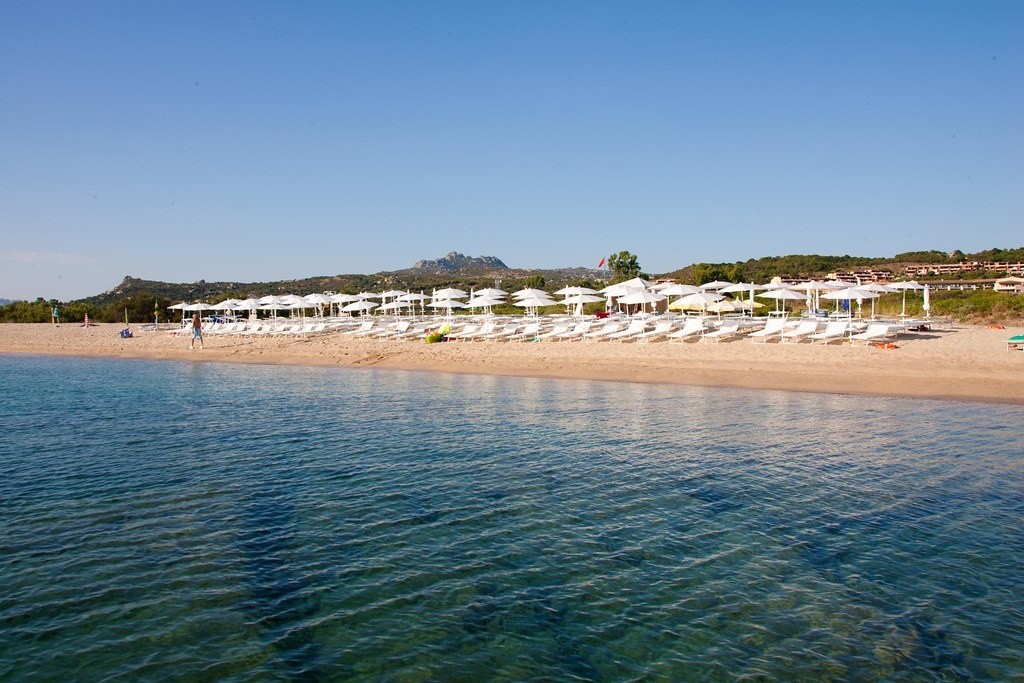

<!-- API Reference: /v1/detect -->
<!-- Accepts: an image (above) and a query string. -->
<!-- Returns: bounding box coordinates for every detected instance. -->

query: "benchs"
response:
[154,310,954,352]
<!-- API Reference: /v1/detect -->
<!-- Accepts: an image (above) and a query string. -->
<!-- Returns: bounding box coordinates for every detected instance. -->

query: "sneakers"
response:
[189,346,194,349]
[199,346,204,349]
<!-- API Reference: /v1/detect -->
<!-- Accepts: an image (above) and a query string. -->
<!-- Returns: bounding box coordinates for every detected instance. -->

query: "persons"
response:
[189,312,205,351]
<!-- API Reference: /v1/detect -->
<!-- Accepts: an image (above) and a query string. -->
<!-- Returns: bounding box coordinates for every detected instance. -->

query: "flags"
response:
[599,257,606,268]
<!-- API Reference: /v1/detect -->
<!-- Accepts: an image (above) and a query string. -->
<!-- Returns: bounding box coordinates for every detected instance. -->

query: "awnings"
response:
[153,299,160,326]
[166,278,936,343]
